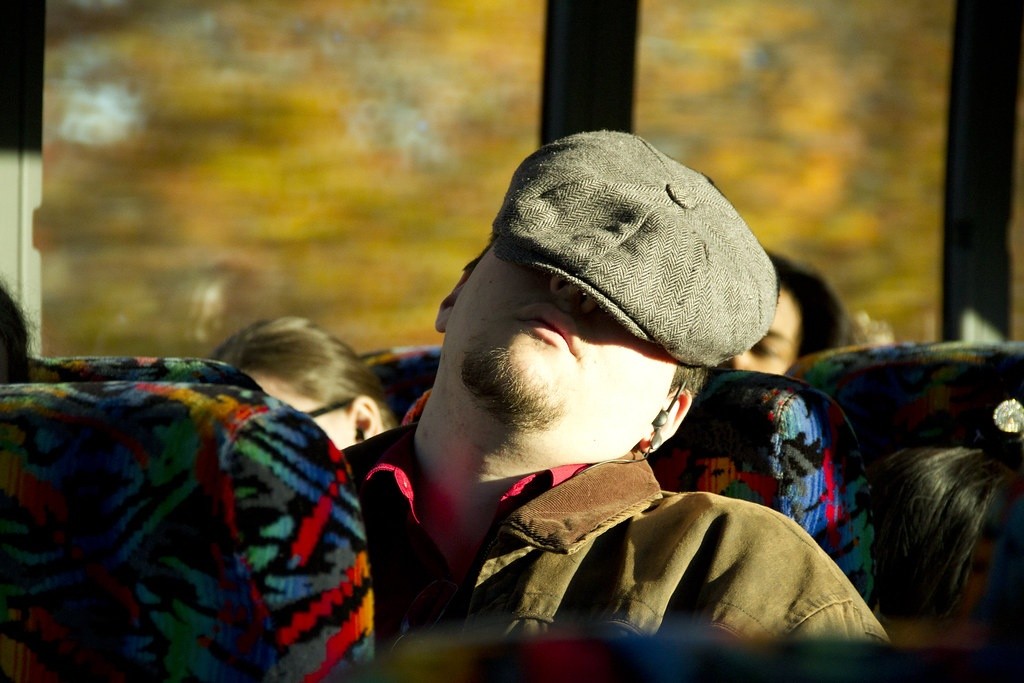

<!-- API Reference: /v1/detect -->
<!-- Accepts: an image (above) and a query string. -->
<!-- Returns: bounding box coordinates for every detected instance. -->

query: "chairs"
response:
[364,346,442,421]
[399,369,878,611]
[0,354,376,682]
[784,340,1023,468]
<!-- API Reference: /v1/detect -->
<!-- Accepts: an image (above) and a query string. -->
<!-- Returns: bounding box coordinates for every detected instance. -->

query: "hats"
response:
[492,129,778,367]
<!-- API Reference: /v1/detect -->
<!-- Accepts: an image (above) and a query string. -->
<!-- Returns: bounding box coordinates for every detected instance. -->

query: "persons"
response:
[875,444,1017,641]
[725,253,851,377]
[339,128,889,653]
[213,314,398,453]
